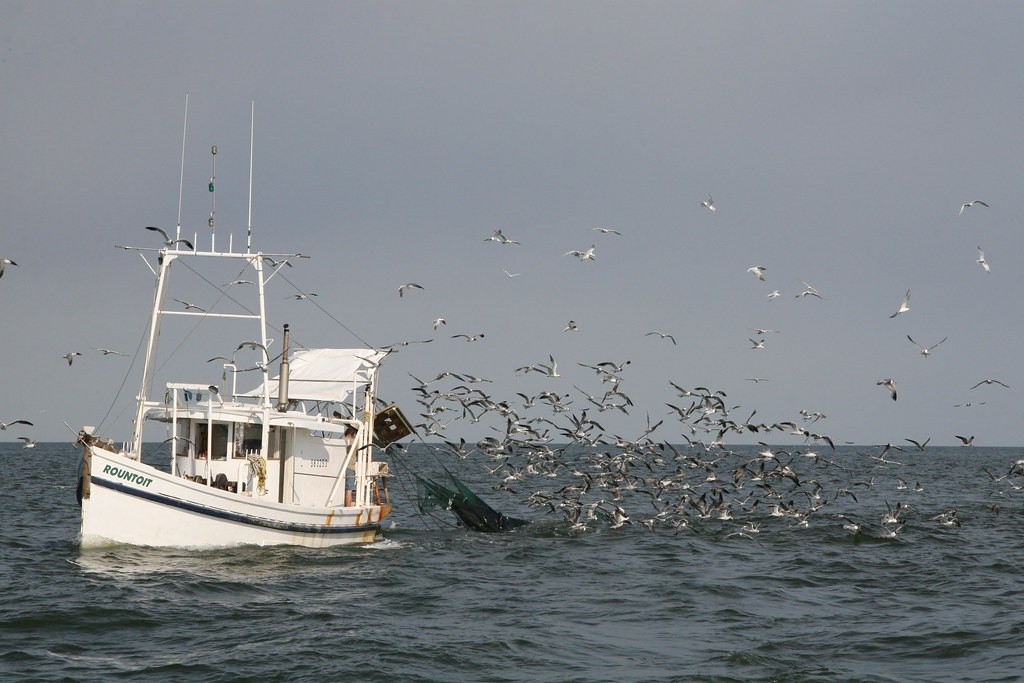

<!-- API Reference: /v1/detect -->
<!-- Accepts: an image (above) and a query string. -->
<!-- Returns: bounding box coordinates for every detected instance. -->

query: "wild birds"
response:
[0,187,1024,530]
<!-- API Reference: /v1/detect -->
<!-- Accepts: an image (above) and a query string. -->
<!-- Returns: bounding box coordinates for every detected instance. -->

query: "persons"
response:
[200,426,214,459]
[341,426,356,507]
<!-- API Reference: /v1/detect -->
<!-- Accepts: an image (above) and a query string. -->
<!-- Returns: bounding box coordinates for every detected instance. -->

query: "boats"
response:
[61,91,420,550]
